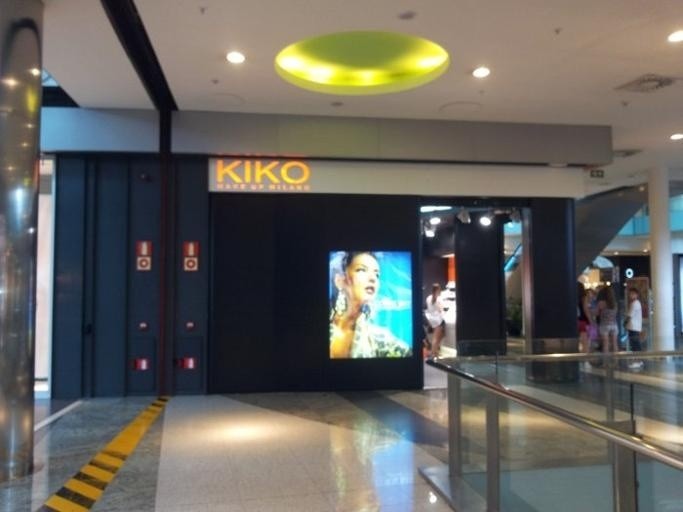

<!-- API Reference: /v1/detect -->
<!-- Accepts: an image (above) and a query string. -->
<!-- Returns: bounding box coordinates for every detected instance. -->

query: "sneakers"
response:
[626,361,644,373]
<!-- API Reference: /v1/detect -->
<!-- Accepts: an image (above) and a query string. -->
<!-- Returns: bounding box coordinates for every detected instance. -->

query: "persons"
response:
[577,281,622,370]
[425,282,448,360]
[331,252,411,358]
[621,287,645,369]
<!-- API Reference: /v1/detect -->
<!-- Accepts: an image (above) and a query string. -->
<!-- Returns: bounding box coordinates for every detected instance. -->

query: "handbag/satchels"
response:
[586,321,599,340]
